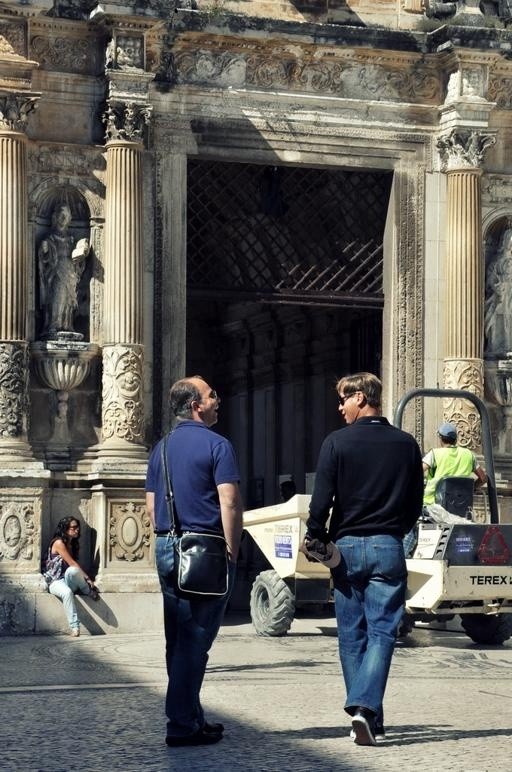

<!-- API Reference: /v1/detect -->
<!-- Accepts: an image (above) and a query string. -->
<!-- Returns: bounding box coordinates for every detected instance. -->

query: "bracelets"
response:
[85,576,92,582]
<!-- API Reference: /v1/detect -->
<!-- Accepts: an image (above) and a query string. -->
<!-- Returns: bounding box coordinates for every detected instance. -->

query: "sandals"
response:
[88,588,101,602]
[71,630,81,638]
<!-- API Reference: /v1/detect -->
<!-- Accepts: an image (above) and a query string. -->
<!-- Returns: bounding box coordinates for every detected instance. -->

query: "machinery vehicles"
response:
[242,389,511,644]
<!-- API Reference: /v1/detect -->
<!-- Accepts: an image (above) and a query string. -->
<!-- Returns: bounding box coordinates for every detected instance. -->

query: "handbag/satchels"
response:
[45,556,64,580]
[170,528,233,605]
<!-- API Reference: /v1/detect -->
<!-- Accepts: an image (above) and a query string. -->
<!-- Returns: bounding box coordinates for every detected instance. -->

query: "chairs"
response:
[435,478,474,522]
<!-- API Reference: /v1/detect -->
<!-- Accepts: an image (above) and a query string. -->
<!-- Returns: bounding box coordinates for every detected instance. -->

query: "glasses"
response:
[334,390,366,404]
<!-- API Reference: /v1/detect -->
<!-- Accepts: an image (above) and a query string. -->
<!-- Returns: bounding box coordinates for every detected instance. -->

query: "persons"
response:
[487,229,511,352]
[303,370,424,747]
[145,376,244,748]
[420,422,489,509]
[45,515,100,638]
[39,200,89,333]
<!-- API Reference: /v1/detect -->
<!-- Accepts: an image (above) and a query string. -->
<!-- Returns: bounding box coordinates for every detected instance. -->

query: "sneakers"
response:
[350,706,386,747]
[163,730,223,747]
[202,722,226,732]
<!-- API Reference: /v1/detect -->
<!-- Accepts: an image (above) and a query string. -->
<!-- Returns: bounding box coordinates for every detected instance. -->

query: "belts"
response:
[153,529,191,539]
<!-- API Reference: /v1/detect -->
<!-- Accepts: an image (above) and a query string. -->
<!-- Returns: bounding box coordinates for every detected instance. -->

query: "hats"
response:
[299,535,342,570]
[436,423,458,438]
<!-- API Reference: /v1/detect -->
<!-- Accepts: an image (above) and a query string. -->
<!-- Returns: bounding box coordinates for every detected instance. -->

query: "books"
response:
[71,237,90,261]
